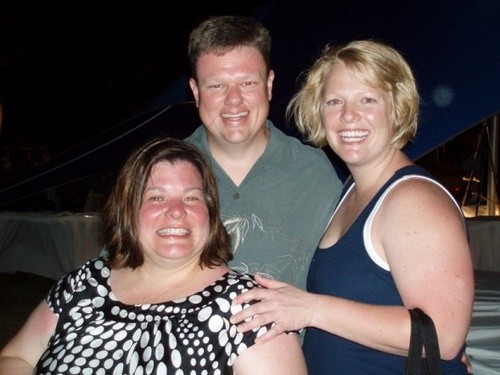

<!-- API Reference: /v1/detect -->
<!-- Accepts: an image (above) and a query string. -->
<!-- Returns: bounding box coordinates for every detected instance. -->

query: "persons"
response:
[179,12,346,349]
[0,137,310,375]
[222,39,477,375]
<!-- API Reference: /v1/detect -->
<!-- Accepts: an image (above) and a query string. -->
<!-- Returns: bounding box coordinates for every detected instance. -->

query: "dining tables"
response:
[0,209,108,282]
